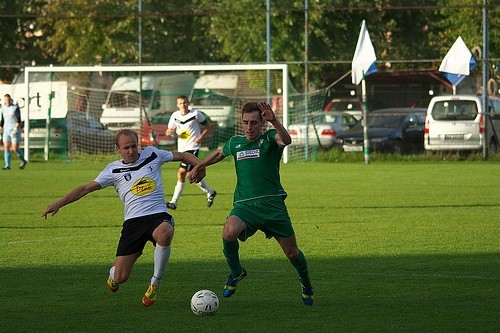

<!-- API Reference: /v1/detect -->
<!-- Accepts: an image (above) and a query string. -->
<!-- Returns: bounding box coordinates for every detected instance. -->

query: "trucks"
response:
[101,73,195,131]
[186,72,263,131]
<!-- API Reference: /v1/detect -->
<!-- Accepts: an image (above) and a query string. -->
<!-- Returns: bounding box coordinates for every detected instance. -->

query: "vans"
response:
[424,95,499,159]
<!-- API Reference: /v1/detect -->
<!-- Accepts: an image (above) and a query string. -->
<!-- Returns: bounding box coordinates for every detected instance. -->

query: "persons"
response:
[188,101,315,306]
[163,95,217,210]
[41,129,207,308]
[0,92,28,171]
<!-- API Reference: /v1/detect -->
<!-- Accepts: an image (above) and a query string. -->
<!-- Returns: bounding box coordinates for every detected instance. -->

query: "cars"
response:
[20,111,118,153]
[139,109,220,149]
[289,112,358,150]
[336,108,427,155]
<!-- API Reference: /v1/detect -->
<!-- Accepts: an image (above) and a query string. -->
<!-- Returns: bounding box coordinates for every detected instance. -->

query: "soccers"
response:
[190,289,218,315]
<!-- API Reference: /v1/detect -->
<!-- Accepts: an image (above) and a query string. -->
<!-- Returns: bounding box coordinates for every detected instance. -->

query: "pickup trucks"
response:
[324,99,368,123]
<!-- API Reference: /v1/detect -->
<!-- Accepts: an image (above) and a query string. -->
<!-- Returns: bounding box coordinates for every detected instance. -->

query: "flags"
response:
[437,35,479,88]
[352,19,379,86]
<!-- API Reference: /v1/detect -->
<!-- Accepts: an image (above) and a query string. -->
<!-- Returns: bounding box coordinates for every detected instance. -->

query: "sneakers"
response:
[223,266,246,297]
[19,161,27,168]
[107,266,119,293]
[206,190,216,207]
[142,284,158,306]
[298,276,314,306]
[2,166,10,170]
[166,202,176,210]
